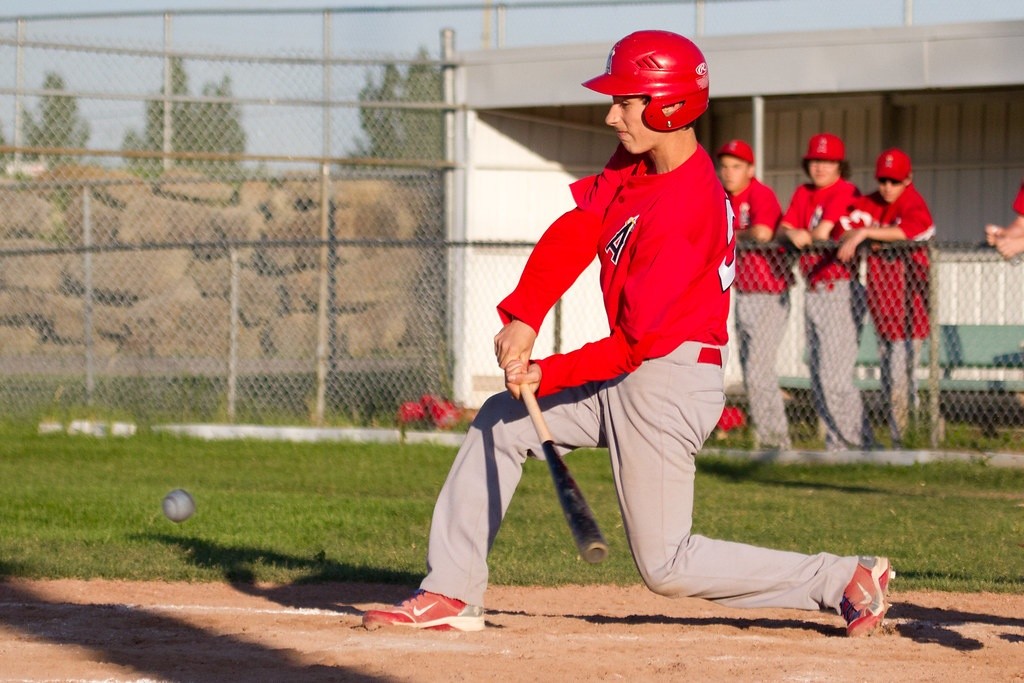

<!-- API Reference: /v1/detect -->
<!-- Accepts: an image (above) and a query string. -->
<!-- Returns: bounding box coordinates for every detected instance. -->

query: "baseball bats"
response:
[519,383,607,565]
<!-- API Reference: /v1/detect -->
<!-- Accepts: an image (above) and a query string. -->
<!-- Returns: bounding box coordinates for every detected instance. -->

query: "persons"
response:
[985,182,1024,262]
[716,138,792,447]
[840,148,935,443]
[364,30,894,637]
[777,131,862,447]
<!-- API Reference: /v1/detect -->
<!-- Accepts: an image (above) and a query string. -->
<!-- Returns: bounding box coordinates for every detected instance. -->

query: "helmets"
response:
[803,132,845,161]
[398,394,462,431]
[581,30,710,132]
[872,148,911,182]
[716,138,754,166]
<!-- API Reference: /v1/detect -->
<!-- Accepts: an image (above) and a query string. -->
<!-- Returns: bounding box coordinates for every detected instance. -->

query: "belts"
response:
[808,281,834,294]
[698,346,722,366]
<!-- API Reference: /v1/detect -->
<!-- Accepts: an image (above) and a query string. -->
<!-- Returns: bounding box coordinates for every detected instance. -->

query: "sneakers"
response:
[362,588,486,632]
[840,556,895,636]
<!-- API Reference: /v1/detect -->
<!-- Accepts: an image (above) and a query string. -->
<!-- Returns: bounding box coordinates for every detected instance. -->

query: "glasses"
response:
[877,176,904,184]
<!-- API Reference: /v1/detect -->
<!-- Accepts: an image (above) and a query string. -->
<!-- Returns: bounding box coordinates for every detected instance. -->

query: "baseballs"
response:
[163,488,196,524]
